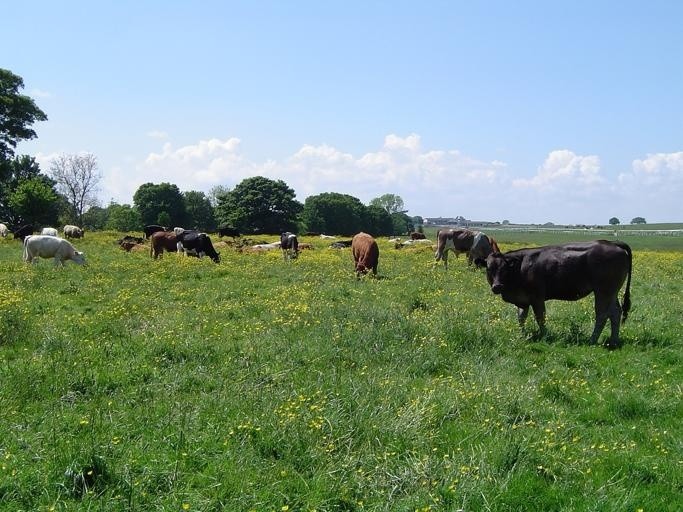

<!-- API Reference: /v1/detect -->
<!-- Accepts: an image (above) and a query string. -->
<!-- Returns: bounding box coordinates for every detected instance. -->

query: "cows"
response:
[490,235,501,254]
[431,227,493,272]
[473,237,634,349]
[0,223,88,269]
[387,232,434,250]
[142,224,384,279]
